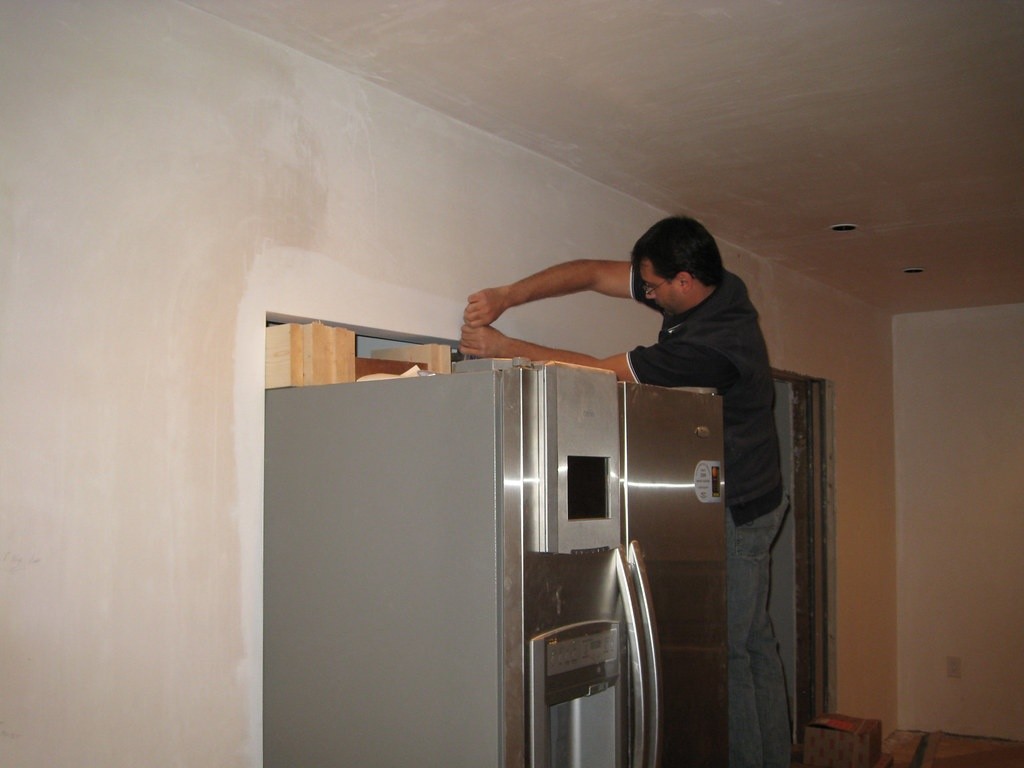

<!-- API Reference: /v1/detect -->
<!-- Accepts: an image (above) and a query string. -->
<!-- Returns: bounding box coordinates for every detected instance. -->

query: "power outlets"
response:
[946,656,963,679]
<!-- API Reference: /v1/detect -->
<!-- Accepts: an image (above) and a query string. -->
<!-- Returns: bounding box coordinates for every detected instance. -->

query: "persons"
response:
[459,215,797,768]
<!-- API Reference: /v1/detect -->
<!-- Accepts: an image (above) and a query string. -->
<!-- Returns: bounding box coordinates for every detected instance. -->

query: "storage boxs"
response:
[803,711,895,768]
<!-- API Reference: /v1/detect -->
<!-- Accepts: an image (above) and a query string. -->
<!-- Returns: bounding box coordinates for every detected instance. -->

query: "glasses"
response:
[642,271,695,295]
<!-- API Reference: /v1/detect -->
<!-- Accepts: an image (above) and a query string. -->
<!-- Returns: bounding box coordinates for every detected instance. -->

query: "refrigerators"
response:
[262,355,734,768]
